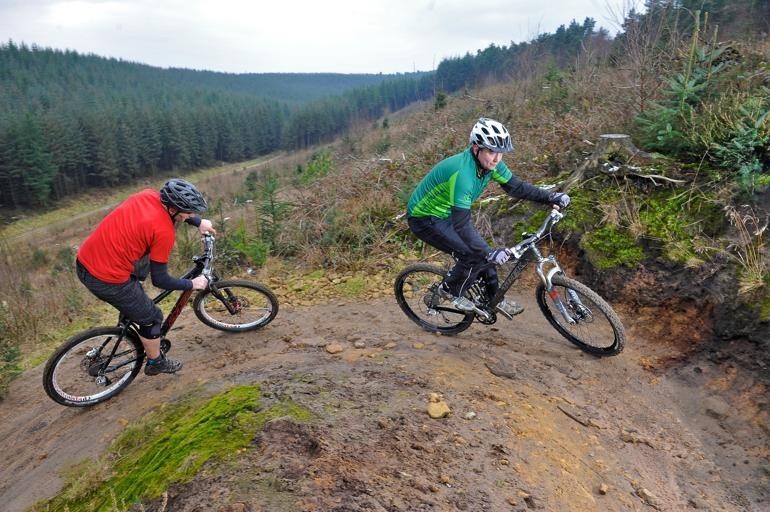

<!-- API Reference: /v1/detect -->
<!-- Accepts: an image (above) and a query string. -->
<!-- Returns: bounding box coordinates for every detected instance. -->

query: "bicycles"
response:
[38,229,279,409]
[391,203,628,359]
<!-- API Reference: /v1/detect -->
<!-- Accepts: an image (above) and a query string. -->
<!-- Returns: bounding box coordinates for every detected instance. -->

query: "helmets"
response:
[469,116,515,153]
[159,178,208,215]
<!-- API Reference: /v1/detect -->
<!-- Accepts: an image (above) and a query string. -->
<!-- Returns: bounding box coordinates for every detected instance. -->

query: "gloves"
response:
[486,247,512,266]
[548,192,571,210]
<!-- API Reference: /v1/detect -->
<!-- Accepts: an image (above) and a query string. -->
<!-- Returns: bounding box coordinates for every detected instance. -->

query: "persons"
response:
[74,176,217,376]
[406,118,571,316]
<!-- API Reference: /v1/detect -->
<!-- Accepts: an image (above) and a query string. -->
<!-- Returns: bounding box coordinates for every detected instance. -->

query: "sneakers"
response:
[496,297,526,315]
[436,283,476,311]
[143,350,183,377]
